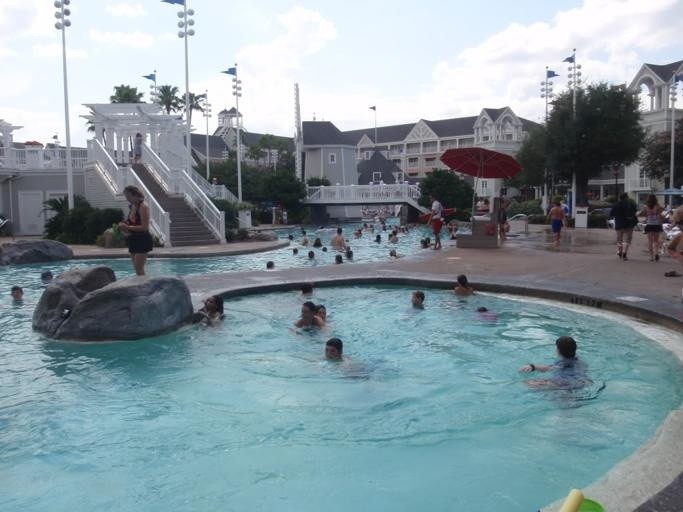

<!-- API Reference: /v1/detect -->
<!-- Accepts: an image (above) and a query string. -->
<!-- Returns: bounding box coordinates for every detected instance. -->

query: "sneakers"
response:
[649,254,660,262]
[617,252,629,261]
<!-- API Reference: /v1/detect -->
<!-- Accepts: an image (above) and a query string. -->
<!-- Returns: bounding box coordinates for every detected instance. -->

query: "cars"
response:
[567,193,683,230]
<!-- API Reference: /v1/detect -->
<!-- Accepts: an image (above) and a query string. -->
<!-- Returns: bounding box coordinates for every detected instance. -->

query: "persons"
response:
[314,305,327,321]
[195,296,224,323]
[636,199,683,258]
[304,337,350,374]
[453,273,473,295]
[10,284,23,301]
[609,192,638,260]
[119,185,152,275]
[518,335,587,399]
[288,301,325,339]
[636,194,663,262]
[410,290,424,308]
[547,200,565,242]
[255,193,511,269]
[40,271,53,281]
[133,132,143,164]
[297,282,313,299]
[475,305,496,324]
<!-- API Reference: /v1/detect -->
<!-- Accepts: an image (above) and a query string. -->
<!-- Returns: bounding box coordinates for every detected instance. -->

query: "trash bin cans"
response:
[238,208,251,229]
[574,207,588,228]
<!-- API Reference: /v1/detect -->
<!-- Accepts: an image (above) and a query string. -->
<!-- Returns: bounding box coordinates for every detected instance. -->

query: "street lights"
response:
[368,105,381,151]
[538,61,557,214]
[564,47,582,219]
[228,63,246,207]
[176,0,196,183]
[199,88,214,184]
[147,67,164,110]
[51,1,78,216]
[667,67,679,210]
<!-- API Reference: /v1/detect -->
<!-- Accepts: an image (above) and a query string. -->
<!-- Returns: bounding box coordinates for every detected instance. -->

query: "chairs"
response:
[605,208,683,264]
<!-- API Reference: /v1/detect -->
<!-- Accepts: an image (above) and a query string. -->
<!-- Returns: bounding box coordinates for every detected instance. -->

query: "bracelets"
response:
[529,362,535,370]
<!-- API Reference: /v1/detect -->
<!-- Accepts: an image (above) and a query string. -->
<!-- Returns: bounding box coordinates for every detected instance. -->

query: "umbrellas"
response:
[439,146,522,192]
[654,186,683,204]
